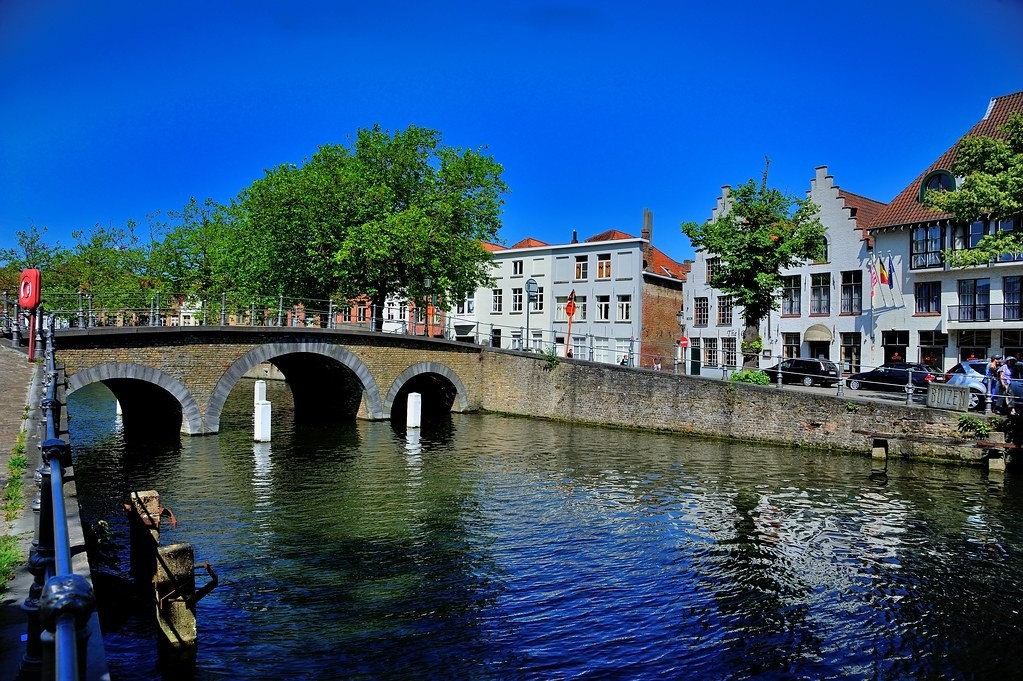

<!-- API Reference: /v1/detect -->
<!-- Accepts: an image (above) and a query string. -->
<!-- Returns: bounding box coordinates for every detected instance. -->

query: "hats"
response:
[1006,356,1017,363]
[991,354,1003,360]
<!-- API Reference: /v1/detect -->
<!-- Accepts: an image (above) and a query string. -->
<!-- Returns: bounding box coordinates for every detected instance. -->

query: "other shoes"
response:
[1011,412,1020,416]
[993,409,1000,413]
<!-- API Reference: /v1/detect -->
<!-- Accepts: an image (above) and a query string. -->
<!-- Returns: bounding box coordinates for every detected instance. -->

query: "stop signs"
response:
[679,336,688,348]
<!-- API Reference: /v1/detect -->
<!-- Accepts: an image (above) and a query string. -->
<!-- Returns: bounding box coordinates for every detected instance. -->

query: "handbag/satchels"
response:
[982,369,994,385]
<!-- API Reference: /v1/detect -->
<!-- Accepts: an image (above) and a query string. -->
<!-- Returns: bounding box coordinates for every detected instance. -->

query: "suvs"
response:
[761,357,841,387]
[944,358,1023,411]
[845,362,943,396]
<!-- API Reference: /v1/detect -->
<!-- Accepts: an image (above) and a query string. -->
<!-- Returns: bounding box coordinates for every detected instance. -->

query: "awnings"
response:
[804,324,832,341]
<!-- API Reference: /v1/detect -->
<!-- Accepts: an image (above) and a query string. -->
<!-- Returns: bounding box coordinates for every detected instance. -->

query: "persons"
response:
[986,351,1023,417]
[620,355,628,366]
[567,349,573,358]
[651,358,661,371]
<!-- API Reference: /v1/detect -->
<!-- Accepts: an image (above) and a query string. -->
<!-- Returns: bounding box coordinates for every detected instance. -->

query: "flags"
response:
[870,265,878,298]
[880,255,888,285]
[888,254,895,290]
